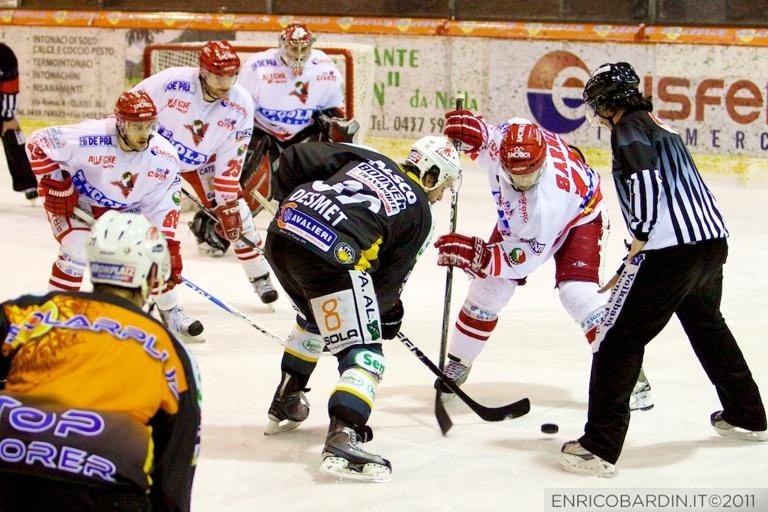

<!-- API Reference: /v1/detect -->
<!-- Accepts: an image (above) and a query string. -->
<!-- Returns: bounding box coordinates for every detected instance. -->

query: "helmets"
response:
[199,40,241,88]
[115,90,157,135]
[500,124,547,187]
[279,25,312,69]
[89,210,172,288]
[583,63,640,127]
[406,137,462,191]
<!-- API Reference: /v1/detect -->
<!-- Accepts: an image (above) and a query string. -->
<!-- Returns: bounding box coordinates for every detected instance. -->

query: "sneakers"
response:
[711,410,740,430]
[562,440,592,455]
[249,272,278,302]
[25,188,38,200]
[434,353,471,393]
[161,305,203,336]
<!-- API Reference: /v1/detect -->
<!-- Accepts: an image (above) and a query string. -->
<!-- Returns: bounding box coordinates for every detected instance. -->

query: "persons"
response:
[558,62,767,478]
[189,22,347,257]
[262,132,461,482]
[130,36,280,315]
[1,42,42,208]
[23,86,206,346]
[1,209,202,512]
[434,109,656,411]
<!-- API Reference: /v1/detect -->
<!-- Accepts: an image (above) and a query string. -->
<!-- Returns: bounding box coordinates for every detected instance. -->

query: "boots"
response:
[324,416,392,474]
[268,370,309,421]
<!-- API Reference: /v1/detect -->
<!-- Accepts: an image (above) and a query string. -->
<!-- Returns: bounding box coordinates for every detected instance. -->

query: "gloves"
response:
[210,199,242,241]
[434,234,492,279]
[445,109,487,153]
[41,171,80,217]
